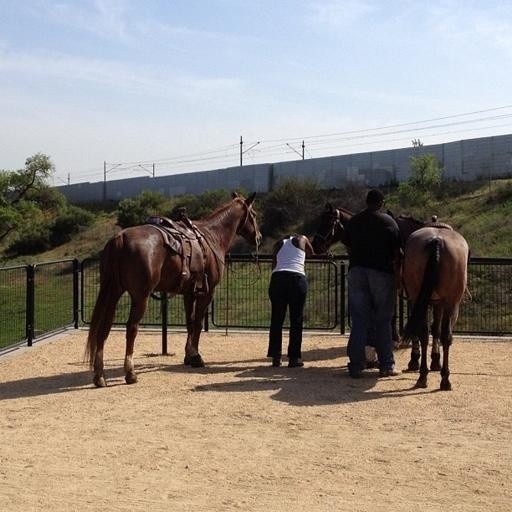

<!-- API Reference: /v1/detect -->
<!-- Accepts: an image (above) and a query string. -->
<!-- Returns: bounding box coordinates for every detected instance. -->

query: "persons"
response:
[344,190,400,378]
[267,234,315,367]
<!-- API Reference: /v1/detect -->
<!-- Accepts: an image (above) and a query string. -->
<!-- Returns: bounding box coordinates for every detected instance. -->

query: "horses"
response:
[386,209,469,392]
[310,201,401,349]
[82,190,263,388]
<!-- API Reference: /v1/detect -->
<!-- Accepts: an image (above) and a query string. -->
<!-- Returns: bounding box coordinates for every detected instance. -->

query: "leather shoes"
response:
[348,370,360,378]
[379,370,402,377]
[288,359,304,367]
[272,359,283,366]
